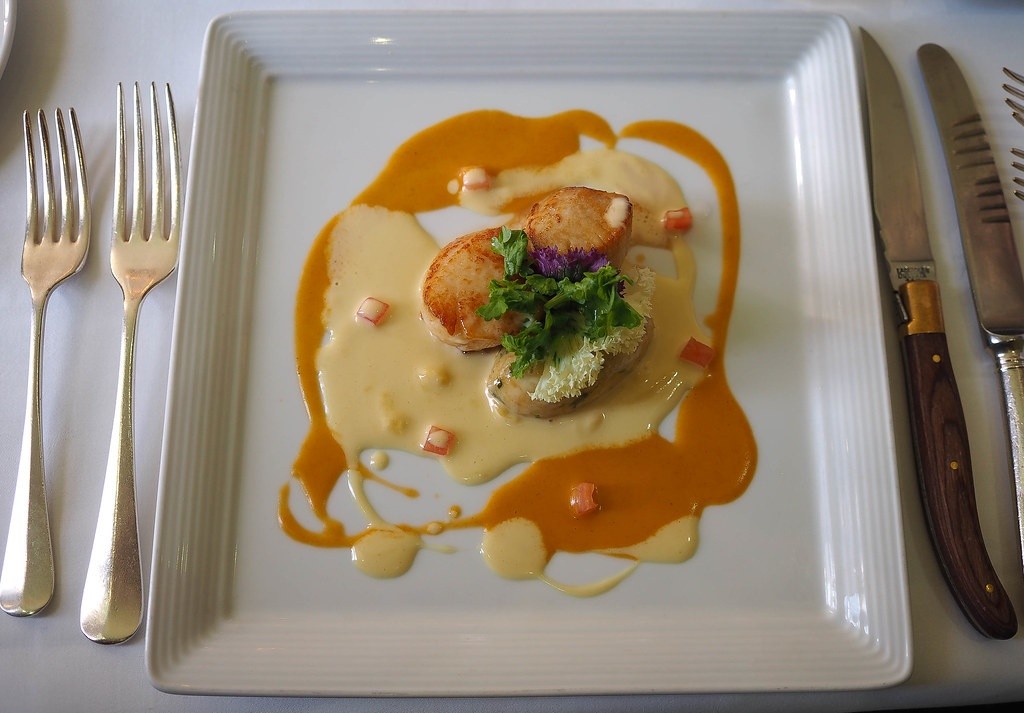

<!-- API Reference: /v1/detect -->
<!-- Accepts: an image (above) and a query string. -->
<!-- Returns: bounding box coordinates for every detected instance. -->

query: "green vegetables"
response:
[473,226,657,404]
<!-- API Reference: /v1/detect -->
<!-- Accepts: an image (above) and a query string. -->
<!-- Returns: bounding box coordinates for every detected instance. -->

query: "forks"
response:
[0,108,91,615]
[1009,148,1024,200]
[1002,67,1024,127]
[80,81,181,644]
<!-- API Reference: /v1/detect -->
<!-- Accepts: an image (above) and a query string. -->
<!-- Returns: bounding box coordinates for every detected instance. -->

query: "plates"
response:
[144,9,914,696]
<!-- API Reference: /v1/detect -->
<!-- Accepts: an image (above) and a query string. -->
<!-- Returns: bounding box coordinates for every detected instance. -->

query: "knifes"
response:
[918,43,1024,560]
[858,26,1019,642]
